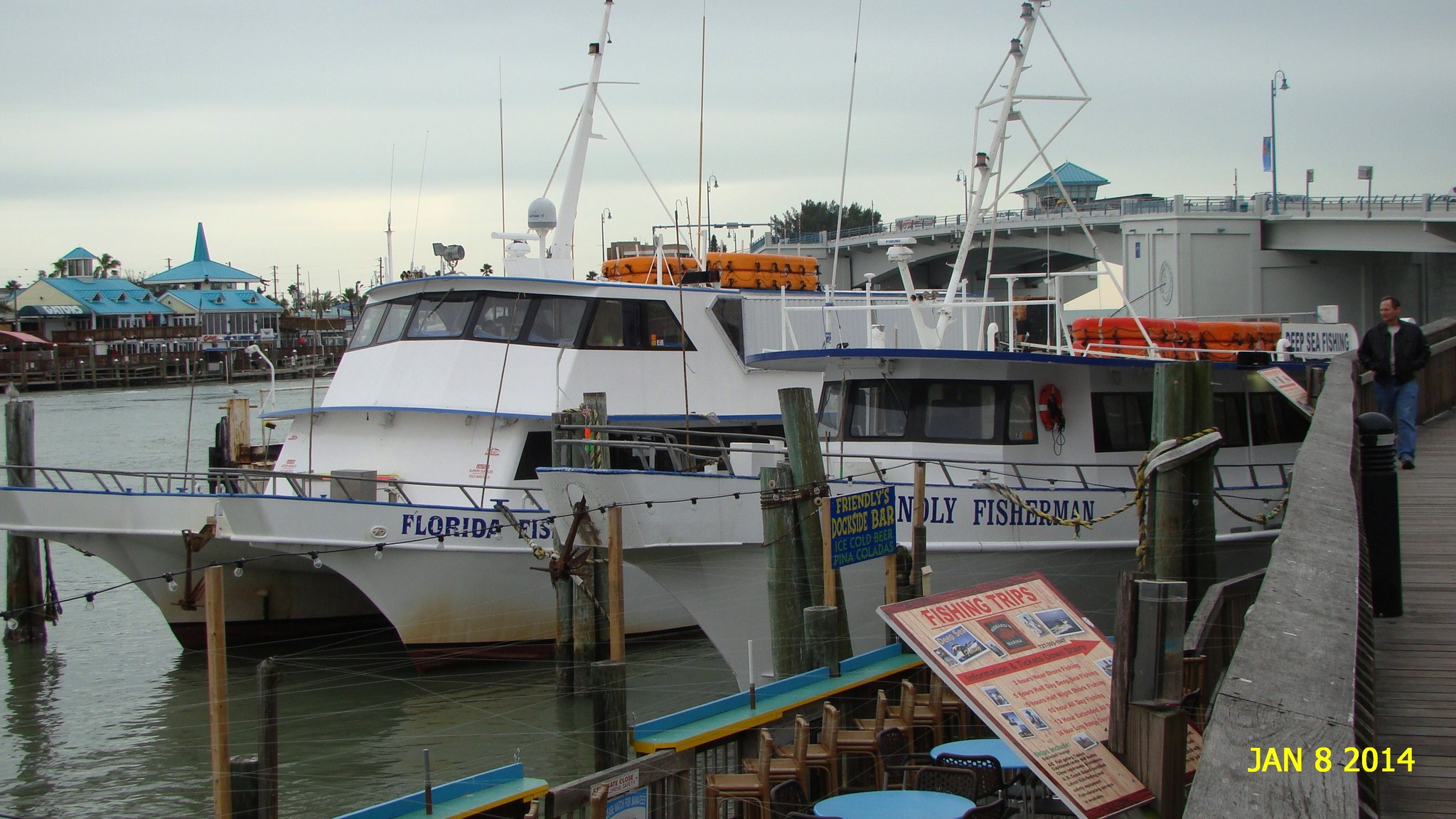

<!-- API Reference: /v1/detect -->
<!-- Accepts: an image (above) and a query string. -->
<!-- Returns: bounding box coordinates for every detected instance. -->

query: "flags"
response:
[147,311,153,321]
[160,313,167,325]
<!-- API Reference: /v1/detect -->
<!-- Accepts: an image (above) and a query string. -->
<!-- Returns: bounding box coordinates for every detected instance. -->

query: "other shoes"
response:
[1401,457,1415,470]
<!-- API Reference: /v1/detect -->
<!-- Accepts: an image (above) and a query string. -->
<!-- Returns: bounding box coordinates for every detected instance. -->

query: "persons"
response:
[1358,297,1431,469]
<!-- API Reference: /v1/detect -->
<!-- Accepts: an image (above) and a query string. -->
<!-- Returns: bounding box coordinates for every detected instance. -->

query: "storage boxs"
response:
[331,469,377,501]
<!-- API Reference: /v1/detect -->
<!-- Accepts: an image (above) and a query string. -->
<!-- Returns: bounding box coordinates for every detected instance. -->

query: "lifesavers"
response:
[897,545,912,582]
[1039,384,1063,430]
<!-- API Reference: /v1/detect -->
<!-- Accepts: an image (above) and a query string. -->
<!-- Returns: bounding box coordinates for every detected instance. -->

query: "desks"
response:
[814,791,976,819]
[930,738,1028,807]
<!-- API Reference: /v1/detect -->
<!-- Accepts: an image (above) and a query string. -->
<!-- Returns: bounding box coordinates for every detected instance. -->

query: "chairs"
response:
[770,728,1074,818]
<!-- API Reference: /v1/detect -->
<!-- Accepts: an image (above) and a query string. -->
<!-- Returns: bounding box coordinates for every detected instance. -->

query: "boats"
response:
[534,0,1307,695]
[0,1,823,663]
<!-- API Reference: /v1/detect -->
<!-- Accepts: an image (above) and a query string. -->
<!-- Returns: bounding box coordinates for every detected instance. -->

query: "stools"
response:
[512,670,968,819]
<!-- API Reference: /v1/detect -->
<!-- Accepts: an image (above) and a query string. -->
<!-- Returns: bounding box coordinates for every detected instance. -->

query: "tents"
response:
[18,305,92,332]
[205,347,246,363]
